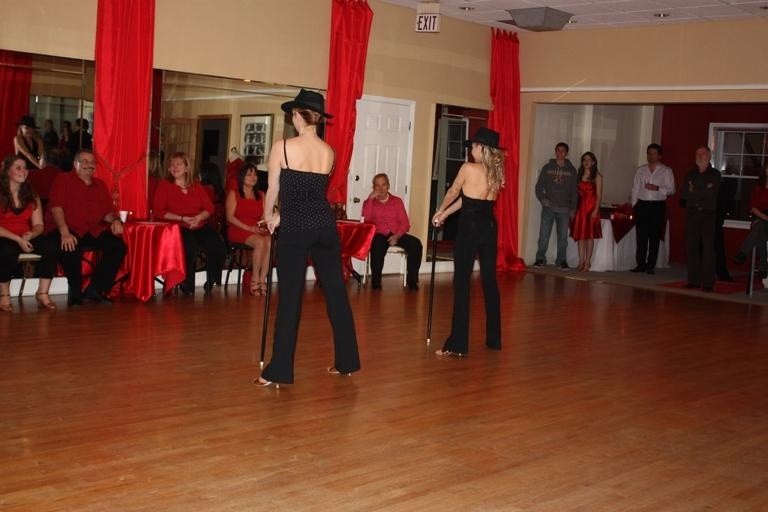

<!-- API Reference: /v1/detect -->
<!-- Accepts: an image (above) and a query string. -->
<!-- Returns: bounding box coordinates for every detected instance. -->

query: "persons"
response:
[568,152,603,273]
[13,114,93,169]
[42,150,127,309]
[361,174,423,291]
[152,152,226,295]
[432,127,508,358]
[0,155,58,312]
[679,145,721,292]
[225,163,271,297]
[732,165,768,279]
[533,142,578,272]
[630,144,676,274]
[251,89,360,388]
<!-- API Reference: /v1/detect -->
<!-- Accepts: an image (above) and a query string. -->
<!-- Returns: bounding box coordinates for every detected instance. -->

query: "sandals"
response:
[577,263,592,272]
[250,280,266,296]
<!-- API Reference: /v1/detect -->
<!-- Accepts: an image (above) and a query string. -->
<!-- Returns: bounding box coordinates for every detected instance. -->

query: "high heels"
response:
[253,377,279,389]
[0,294,12,311]
[36,292,55,309]
[327,367,351,376]
[435,349,461,356]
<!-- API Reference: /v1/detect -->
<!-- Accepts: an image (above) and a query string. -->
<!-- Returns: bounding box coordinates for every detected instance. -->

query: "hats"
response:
[281,89,333,118]
[464,127,502,149]
[16,116,40,129]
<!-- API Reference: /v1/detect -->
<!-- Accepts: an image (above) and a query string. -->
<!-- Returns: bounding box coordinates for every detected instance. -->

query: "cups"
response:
[119,211,128,223]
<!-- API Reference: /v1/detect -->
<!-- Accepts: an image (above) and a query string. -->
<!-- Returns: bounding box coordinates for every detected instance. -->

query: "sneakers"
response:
[533,260,546,270]
[557,263,568,270]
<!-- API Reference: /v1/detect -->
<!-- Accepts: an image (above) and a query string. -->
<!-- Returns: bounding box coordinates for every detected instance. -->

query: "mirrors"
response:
[0,47,326,269]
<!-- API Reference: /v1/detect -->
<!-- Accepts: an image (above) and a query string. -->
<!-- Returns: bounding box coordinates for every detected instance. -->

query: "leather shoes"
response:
[68,285,111,307]
[407,282,419,292]
[181,281,213,294]
[703,253,745,292]
[631,265,654,274]
[372,280,381,291]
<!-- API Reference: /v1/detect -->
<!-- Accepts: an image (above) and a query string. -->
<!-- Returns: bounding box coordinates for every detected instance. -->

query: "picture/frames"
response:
[238,112,277,169]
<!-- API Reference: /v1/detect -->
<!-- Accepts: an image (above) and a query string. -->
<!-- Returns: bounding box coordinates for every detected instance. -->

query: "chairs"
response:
[68,245,105,305]
[222,241,256,296]
[17,254,48,298]
[362,246,410,287]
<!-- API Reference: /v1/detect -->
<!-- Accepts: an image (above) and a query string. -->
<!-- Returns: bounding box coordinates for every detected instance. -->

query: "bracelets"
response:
[656,186,658,191]
[181,216,183,222]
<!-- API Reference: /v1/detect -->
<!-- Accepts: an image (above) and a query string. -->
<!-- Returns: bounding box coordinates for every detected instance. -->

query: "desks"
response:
[116,220,187,303]
[309,219,376,289]
[566,209,670,272]
